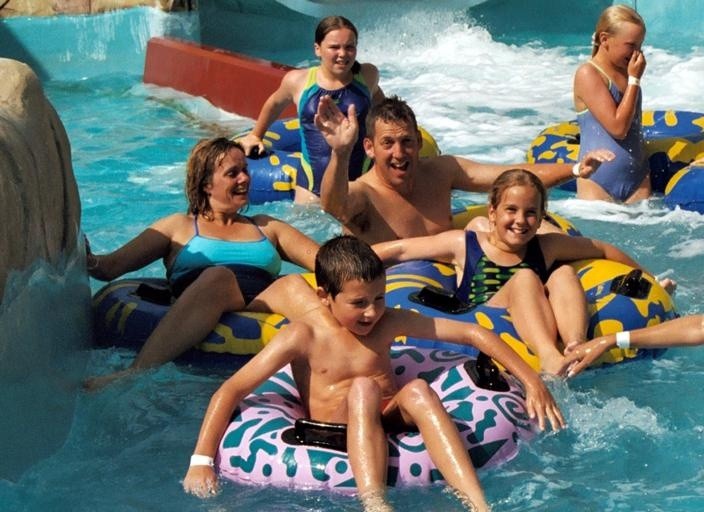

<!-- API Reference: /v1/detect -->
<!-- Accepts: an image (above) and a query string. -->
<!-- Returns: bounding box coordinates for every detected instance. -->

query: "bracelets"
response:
[615,330,631,350]
[189,454,215,468]
[572,161,582,178]
[86,254,100,270]
[627,75,640,87]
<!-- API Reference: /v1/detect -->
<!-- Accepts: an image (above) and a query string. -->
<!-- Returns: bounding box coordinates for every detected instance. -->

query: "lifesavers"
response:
[214,345,542,494]
[386,256,676,371]
[226,115,440,203]
[91,272,317,373]
[528,108,704,214]
[448,203,586,241]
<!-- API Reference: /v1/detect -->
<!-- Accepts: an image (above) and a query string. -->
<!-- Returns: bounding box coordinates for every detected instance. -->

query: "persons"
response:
[313,92,678,297]
[81,137,325,397]
[555,313,703,379]
[231,15,385,198]
[574,4,652,207]
[369,168,658,379]
[182,234,569,512]
